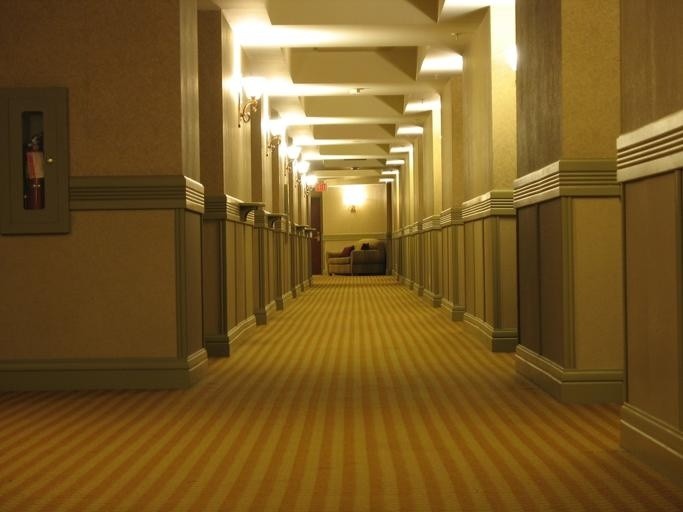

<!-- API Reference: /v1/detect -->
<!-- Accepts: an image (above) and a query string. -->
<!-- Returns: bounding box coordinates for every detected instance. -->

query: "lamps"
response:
[240,75,302,175]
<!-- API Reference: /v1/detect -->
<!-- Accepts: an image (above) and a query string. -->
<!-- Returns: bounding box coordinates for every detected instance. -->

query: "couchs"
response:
[327,240,385,276]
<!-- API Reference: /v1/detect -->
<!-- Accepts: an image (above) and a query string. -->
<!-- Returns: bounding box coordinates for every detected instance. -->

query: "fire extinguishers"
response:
[24,133,44,209]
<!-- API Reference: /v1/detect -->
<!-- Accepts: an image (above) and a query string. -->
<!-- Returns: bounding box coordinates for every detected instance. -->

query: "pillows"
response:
[339,243,369,257]
